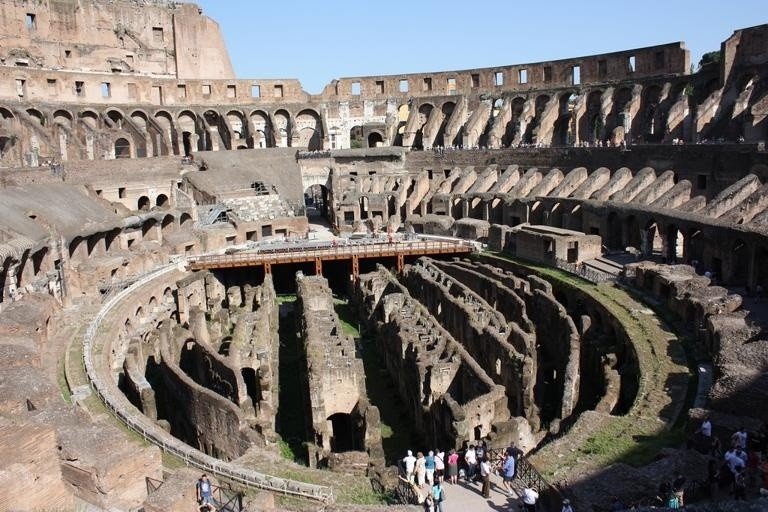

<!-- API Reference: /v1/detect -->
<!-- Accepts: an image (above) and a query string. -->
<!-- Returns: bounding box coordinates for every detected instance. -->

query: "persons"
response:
[523,485,540,512]
[672,136,745,145]
[196,502,217,512]
[571,260,579,271]
[603,428,767,512]
[196,473,214,504]
[428,144,505,149]
[512,141,552,148]
[560,498,573,512]
[692,415,712,442]
[573,136,641,148]
[402,440,524,512]
[646,249,767,306]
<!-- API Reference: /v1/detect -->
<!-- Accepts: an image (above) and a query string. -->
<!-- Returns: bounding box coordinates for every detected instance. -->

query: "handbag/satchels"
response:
[439,489,445,502]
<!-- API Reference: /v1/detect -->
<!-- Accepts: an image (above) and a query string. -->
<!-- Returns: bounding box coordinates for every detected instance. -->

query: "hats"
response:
[407,449,456,458]
